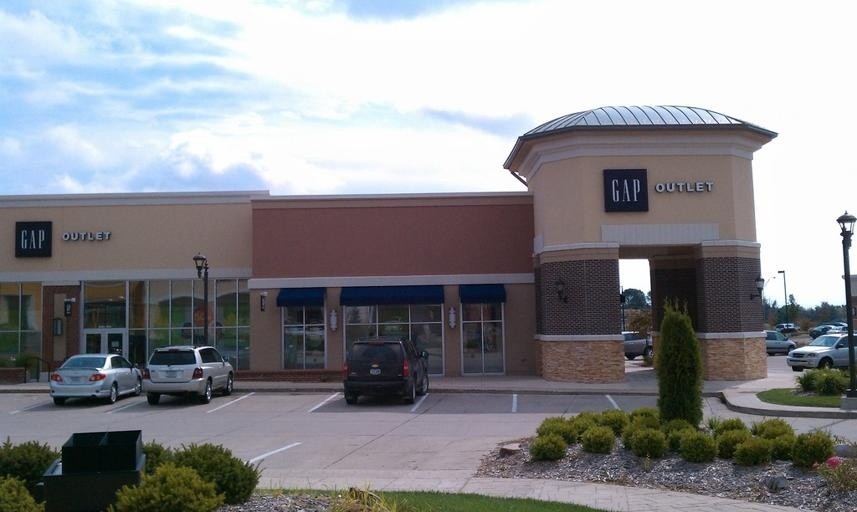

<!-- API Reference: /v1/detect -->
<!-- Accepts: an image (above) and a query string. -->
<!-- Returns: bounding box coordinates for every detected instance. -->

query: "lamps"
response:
[53,319,62,336]
[555,276,568,303]
[750,273,764,299]
[330,309,338,332]
[448,307,457,329]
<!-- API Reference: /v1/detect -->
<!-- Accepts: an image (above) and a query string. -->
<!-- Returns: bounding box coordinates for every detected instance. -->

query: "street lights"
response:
[837,210,856,401]
[192,253,209,345]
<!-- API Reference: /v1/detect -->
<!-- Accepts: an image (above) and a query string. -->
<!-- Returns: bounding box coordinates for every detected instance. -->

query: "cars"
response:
[766,320,855,375]
[342,335,430,406]
[49,353,144,405]
[143,343,235,406]
[621,329,653,359]
